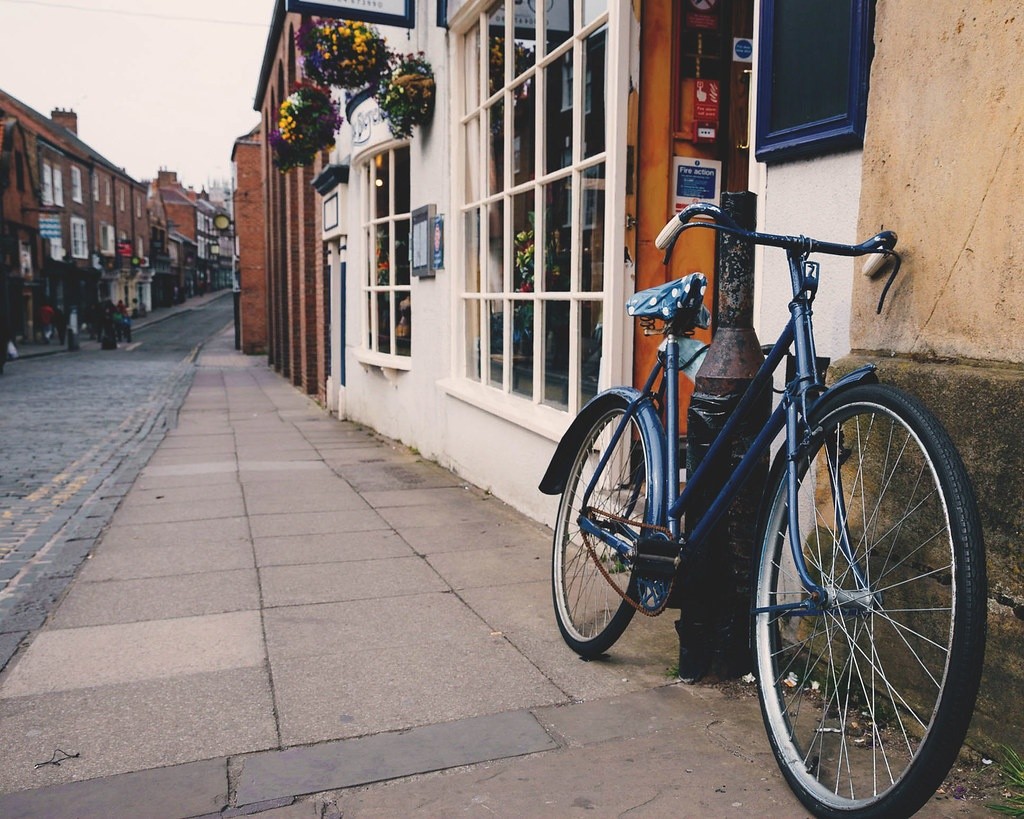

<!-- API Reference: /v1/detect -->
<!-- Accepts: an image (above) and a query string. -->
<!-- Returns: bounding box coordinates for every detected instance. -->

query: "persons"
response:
[85,299,132,344]
[38,294,67,347]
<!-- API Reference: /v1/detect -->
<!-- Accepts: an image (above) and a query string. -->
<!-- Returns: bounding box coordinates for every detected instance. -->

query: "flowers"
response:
[488,34,530,113]
[264,81,343,171]
[295,16,391,89]
[376,54,440,140]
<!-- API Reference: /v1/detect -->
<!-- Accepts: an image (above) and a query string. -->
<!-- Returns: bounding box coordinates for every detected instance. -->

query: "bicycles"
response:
[538,202,988,818]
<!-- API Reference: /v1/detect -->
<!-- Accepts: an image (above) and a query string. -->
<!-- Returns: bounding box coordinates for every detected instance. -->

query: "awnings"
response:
[175,230,198,250]
[167,233,185,244]
